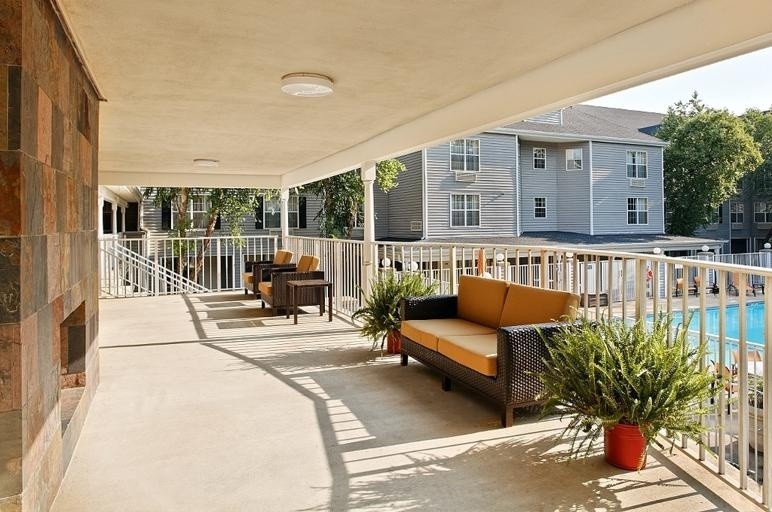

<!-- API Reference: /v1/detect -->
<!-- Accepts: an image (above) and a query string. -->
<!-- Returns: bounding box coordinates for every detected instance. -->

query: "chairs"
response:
[676,273,756,296]
[243,249,324,313]
[708,359,744,416]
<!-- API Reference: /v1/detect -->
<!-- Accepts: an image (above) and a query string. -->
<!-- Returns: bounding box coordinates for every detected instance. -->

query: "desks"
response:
[284,278,333,323]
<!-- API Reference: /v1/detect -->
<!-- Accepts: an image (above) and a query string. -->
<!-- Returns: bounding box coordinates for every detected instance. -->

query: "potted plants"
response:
[352,269,440,353]
[742,371,764,454]
[528,302,736,473]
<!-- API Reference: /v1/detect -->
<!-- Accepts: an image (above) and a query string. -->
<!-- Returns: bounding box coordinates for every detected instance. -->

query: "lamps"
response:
[279,73,337,101]
[193,159,221,166]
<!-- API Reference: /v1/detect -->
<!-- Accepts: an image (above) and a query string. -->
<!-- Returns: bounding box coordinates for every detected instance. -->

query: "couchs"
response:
[396,272,580,426]
